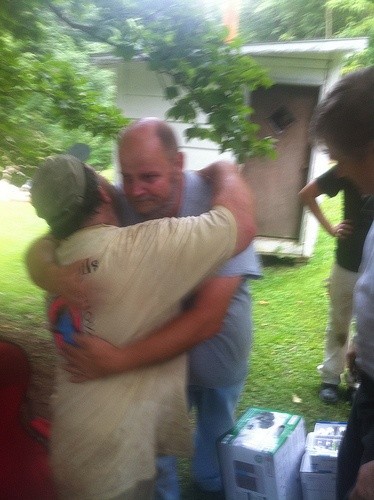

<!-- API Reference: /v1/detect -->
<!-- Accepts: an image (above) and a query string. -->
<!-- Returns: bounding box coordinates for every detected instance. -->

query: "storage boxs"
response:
[218,409,348,500]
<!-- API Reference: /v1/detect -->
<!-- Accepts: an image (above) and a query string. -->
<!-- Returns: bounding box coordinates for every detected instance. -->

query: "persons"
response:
[297,65,374,500]
[23,117,264,500]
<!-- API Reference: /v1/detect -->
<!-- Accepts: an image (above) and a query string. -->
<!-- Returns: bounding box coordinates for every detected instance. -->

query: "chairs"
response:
[0,341,60,500]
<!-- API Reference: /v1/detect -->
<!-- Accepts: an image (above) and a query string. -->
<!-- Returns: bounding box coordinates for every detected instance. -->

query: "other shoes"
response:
[319,383,338,402]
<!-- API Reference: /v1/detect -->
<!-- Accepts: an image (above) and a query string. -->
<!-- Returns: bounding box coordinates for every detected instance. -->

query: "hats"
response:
[30,154,85,228]
